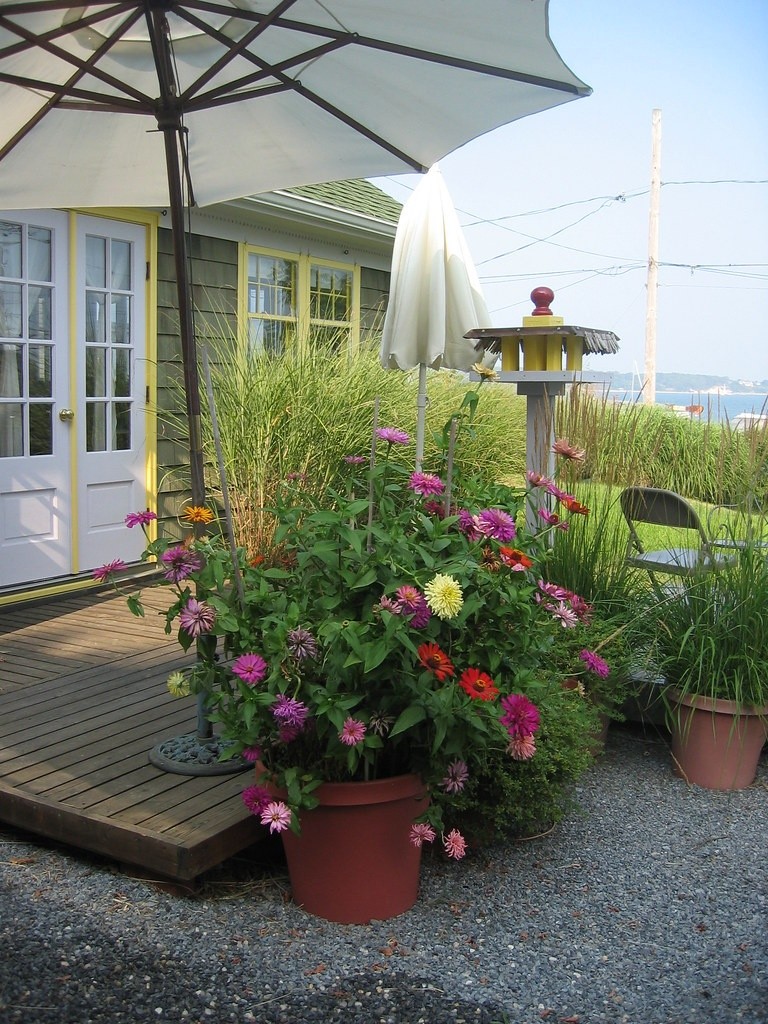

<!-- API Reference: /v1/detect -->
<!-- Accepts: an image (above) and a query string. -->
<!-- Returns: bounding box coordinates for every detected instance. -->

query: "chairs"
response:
[617,489,740,608]
[706,490,768,549]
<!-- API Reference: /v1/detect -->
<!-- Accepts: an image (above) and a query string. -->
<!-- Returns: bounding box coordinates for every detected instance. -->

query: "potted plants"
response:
[519,490,768,791]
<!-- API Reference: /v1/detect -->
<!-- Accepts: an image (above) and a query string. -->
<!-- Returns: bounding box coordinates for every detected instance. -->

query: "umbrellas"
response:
[380,160,492,498]
[0,0,597,741]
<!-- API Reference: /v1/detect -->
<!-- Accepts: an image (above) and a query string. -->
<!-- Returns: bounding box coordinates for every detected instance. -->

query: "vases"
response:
[252,773,430,924]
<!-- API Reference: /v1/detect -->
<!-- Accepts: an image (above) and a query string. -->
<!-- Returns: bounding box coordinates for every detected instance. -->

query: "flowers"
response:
[98,425,610,863]
